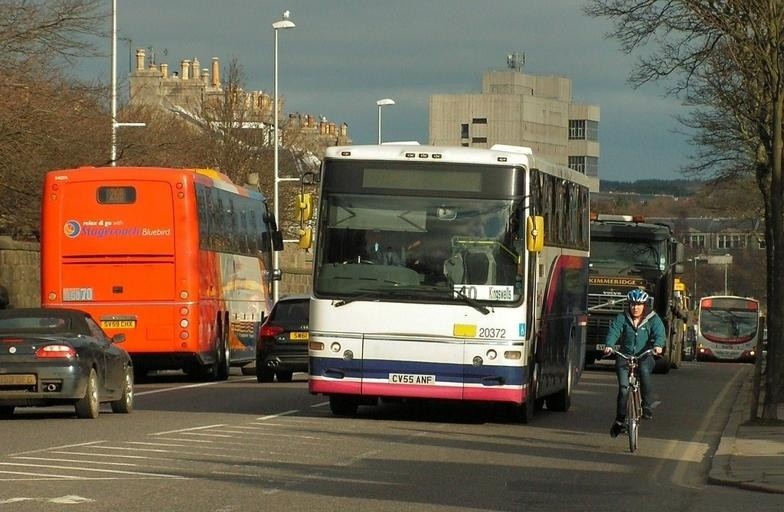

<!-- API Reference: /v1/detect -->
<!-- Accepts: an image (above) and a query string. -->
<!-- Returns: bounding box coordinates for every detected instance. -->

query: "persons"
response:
[604,289,666,438]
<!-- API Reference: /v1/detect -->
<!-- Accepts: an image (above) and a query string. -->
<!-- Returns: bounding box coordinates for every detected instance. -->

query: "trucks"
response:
[587,209,698,375]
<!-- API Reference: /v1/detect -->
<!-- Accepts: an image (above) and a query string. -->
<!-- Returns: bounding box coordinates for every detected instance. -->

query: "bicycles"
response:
[598,348,665,453]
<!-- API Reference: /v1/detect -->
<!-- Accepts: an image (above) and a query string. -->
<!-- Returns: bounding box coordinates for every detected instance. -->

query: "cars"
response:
[0,304,138,421]
[762,329,768,352]
[256,295,310,386]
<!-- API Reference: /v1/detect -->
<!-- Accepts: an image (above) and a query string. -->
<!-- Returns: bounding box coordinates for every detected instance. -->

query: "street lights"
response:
[376,96,397,145]
[270,17,303,313]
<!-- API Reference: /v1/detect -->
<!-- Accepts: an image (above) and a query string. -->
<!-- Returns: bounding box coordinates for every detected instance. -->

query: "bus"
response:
[695,294,762,364]
[38,162,286,386]
[290,135,595,427]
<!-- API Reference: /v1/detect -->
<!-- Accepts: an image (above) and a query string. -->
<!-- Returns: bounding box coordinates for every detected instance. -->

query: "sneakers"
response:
[609,422,626,439]
[640,407,653,419]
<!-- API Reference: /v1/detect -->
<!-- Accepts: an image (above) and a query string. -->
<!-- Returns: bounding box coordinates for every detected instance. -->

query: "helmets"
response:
[626,288,649,306]
